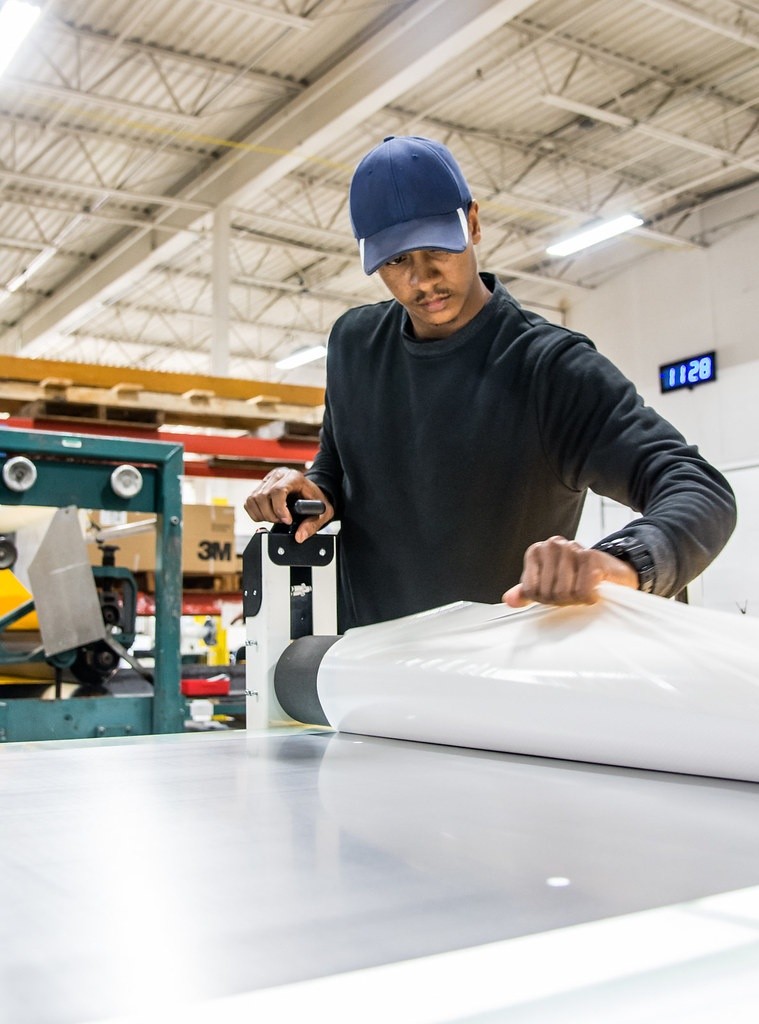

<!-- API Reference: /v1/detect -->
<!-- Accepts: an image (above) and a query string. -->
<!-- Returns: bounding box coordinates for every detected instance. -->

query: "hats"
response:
[349,135,472,275]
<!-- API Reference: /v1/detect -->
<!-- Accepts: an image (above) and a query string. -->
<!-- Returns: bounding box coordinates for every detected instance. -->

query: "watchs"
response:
[596,536,654,594]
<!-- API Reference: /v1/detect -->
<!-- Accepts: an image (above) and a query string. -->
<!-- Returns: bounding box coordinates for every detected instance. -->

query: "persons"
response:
[243,134,737,636]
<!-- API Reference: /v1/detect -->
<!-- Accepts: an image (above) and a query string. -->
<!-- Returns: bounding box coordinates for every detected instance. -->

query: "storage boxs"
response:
[88,505,236,573]
